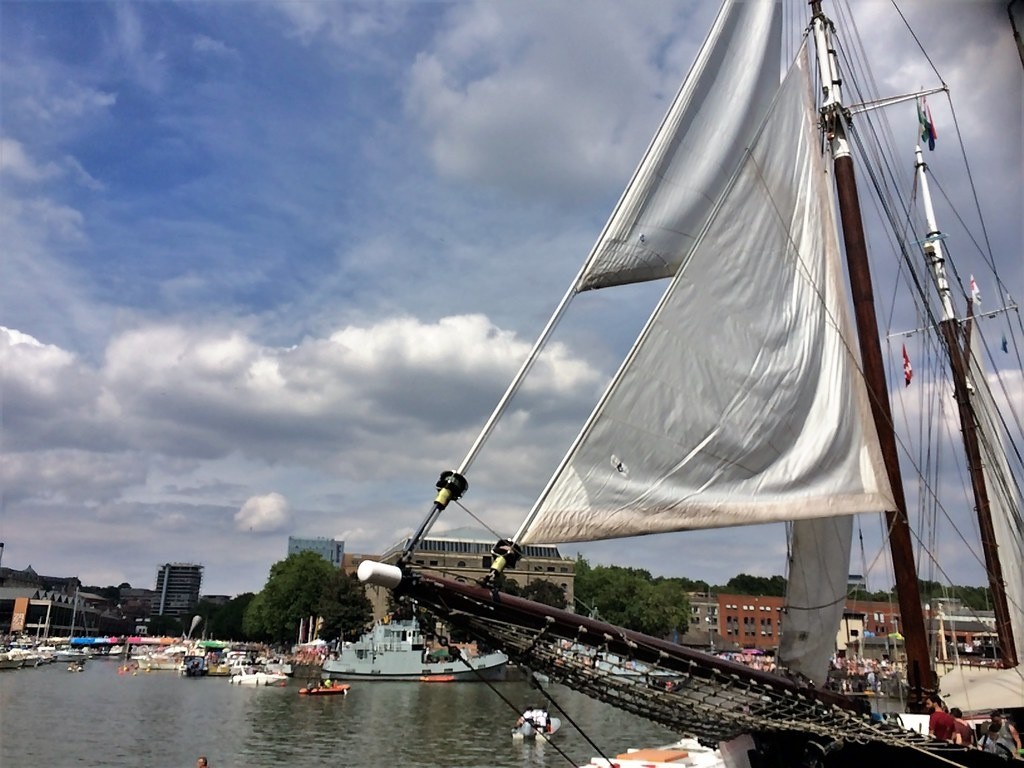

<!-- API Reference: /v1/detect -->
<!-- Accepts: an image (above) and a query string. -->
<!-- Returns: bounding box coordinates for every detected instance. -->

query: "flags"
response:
[1001,335,1008,353]
[916,93,937,150]
[902,344,912,387]
[970,274,981,305]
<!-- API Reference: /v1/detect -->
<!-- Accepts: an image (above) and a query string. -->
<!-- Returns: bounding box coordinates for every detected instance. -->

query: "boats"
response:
[232,672,288,686]
[513,718,561,739]
[299,681,350,694]
[0,639,122,670]
[131,646,296,675]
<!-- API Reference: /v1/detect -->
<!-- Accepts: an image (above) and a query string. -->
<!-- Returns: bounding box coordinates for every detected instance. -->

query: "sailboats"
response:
[355,0,1024,767]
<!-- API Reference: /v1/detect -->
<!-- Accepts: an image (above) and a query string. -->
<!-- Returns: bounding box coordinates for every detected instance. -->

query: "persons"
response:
[319,678,332,688]
[924,694,956,744]
[978,724,1008,757]
[197,756,210,768]
[950,706,974,747]
[306,681,312,691]
[522,706,551,733]
[991,712,1022,758]
[246,651,279,665]
[715,654,907,692]
